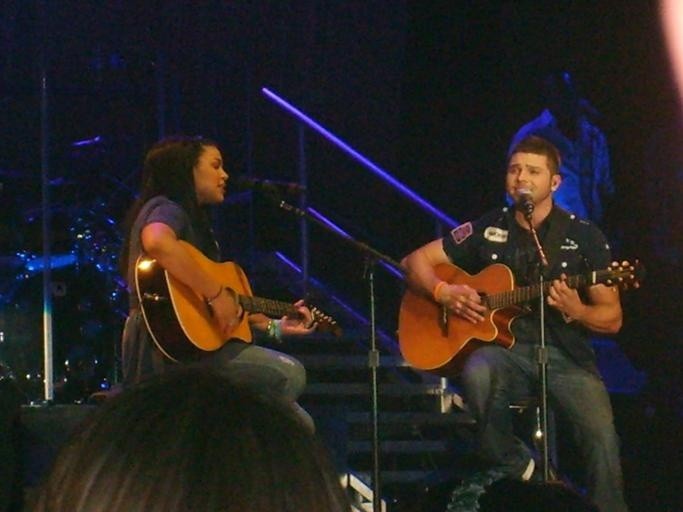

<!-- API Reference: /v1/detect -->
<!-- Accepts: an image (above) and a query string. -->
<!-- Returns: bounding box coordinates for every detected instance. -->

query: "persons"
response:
[504,65,616,229]
[118,130,317,449]
[401,135,626,511]
[27,367,353,511]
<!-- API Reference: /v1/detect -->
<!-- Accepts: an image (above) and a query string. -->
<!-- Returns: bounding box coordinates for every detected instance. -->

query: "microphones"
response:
[230,172,309,199]
[516,188,535,213]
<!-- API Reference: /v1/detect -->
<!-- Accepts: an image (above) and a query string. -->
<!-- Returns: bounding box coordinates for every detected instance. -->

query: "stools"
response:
[506,394,557,482]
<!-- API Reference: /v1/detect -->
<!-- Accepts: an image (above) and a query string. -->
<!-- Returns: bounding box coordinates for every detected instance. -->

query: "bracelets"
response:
[206,284,224,304]
[265,318,282,339]
[431,281,448,304]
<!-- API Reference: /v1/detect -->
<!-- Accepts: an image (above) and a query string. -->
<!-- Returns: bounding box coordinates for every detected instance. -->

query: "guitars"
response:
[134,240,344,363]
[399,259,645,375]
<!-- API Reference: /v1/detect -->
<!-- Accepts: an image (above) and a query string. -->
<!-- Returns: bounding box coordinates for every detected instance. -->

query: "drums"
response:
[0,257,131,407]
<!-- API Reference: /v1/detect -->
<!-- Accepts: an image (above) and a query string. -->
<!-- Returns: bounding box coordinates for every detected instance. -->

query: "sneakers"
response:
[519,458,536,480]
